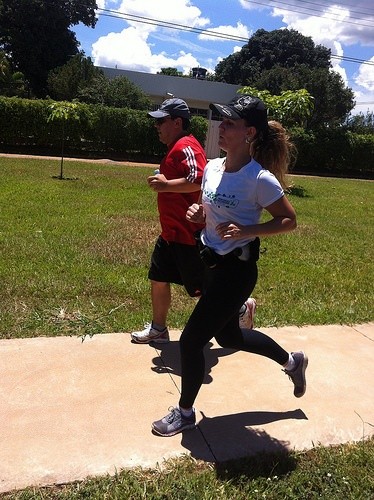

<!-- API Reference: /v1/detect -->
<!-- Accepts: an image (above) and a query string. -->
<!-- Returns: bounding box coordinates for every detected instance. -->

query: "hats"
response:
[146,99,191,119]
[210,95,267,124]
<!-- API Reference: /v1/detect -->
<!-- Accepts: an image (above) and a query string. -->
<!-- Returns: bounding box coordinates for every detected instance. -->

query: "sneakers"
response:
[239,298,255,330]
[151,406,196,436]
[281,351,308,398]
[131,321,169,343]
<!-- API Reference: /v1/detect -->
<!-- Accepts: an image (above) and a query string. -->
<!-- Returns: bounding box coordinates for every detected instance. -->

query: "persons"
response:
[152,95,309,438]
[128,98,257,345]
